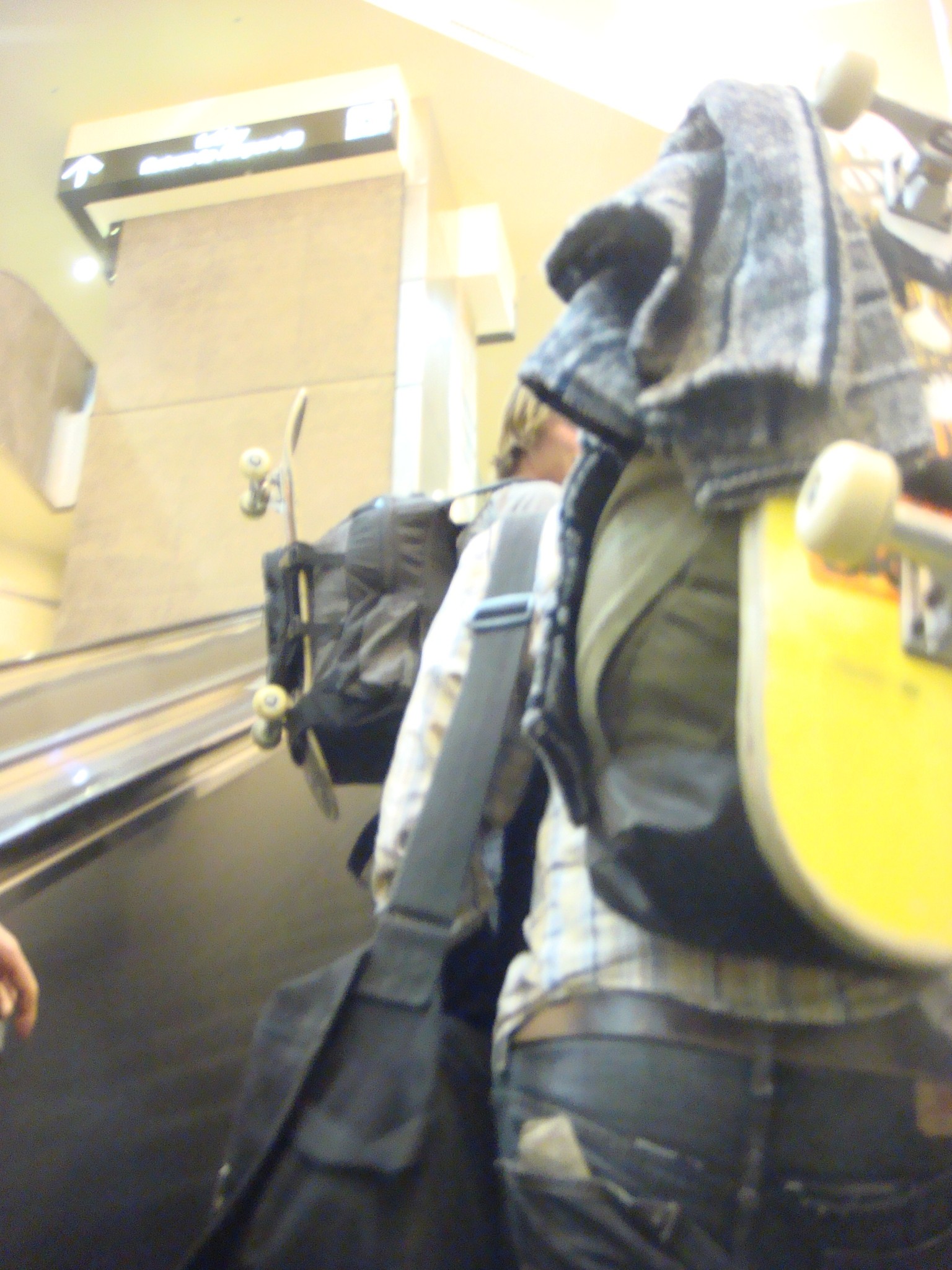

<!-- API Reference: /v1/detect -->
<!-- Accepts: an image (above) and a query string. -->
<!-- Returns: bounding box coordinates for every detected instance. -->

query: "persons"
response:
[492,19,952,1270]
[0,924,40,1037]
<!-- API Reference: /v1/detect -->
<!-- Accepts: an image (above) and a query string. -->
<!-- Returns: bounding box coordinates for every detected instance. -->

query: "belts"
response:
[509,993,951,1079]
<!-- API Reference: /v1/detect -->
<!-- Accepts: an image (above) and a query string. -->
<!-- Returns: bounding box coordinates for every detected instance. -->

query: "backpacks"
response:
[265,476,537,787]
[536,438,856,962]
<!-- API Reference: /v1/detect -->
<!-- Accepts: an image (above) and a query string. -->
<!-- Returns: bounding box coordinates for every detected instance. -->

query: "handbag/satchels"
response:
[181,943,516,1269]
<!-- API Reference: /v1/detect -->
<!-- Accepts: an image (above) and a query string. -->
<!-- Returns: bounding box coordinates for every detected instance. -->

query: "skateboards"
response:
[738,53,952,973]
[240,390,335,819]
